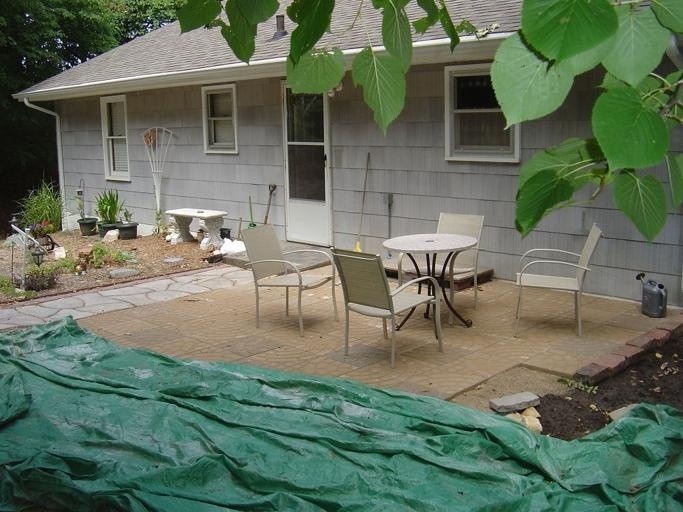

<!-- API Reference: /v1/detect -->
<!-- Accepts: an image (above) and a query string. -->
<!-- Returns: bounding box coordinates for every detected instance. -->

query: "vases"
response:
[35,233,47,239]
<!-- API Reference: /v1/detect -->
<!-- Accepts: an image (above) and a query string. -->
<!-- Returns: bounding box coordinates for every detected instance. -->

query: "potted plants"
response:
[76,197,98,235]
[104,190,122,239]
[96,189,111,238]
[118,208,138,240]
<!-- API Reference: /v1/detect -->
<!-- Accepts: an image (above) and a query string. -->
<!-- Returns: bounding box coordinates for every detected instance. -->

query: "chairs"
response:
[513,222,602,337]
[331,247,444,369]
[240,224,339,337]
[398,213,484,326]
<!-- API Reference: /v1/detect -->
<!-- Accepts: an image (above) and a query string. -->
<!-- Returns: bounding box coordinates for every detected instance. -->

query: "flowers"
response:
[28,220,54,235]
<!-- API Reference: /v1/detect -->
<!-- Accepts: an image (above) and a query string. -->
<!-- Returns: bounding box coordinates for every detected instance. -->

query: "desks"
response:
[382,234,477,328]
